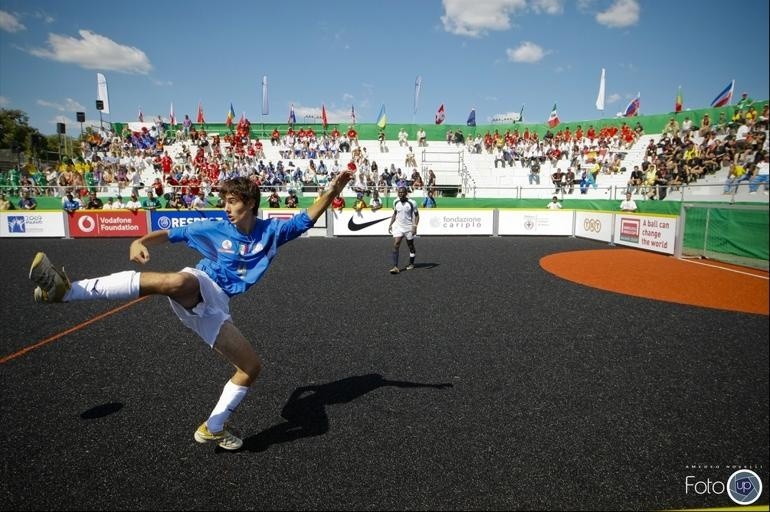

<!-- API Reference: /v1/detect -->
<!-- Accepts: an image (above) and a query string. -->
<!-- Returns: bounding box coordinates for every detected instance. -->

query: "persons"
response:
[1,117,437,215]
[26,170,353,451]
[388,186,419,273]
[449,105,769,210]
[736,91,756,115]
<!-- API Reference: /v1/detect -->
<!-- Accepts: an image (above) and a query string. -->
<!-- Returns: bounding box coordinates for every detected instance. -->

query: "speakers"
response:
[57,123,65,134]
[96,100,103,110]
[77,112,85,122]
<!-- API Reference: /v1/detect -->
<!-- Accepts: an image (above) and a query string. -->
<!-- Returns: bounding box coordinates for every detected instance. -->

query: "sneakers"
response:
[389,264,415,275]
[28,247,69,309]
[193,423,245,452]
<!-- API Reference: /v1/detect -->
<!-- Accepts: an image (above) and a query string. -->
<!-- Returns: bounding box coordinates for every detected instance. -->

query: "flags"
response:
[710,79,735,108]
[616,91,641,118]
[414,74,421,113]
[376,103,387,131]
[674,84,682,113]
[136,100,246,128]
[594,68,605,111]
[351,103,356,124]
[288,102,298,126]
[435,103,445,125]
[320,104,327,127]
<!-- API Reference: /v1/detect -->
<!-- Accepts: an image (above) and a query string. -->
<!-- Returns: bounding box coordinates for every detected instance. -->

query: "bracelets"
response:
[413,224,418,227]
